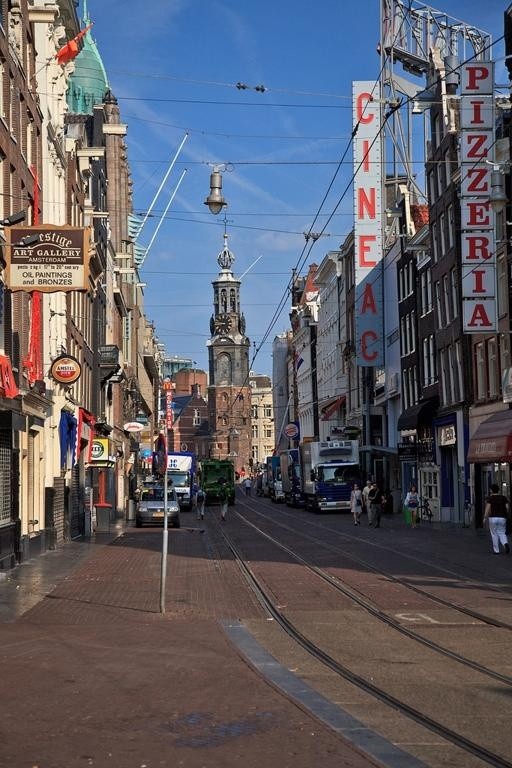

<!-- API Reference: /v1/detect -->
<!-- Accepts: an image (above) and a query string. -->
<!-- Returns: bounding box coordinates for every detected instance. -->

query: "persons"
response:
[403,485,420,529]
[482,484,510,555]
[217,480,231,522]
[363,480,374,526]
[350,483,364,526]
[191,481,207,521]
[243,477,252,497]
[367,482,387,528]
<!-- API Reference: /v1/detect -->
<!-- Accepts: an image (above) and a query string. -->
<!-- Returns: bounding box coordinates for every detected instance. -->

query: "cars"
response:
[136,486,180,530]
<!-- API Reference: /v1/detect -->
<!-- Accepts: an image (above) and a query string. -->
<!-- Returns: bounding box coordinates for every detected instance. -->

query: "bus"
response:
[201,459,236,506]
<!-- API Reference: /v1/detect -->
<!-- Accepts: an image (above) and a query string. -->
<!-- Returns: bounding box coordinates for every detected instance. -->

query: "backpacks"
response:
[219,488,227,501]
[197,492,204,503]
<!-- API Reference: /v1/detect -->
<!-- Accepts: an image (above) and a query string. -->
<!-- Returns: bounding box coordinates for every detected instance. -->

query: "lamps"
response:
[484,161,512,214]
[0,211,26,226]
[0,234,39,247]
[204,163,227,215]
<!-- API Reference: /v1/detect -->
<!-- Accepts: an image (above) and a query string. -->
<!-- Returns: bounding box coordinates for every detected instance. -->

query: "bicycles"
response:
[417,494,432,523]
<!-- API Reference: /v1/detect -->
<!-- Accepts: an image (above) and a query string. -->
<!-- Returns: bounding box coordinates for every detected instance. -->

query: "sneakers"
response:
[504,543,510,554]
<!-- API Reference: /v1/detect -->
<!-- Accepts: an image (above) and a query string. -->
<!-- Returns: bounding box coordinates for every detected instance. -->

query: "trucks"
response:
[266,449,300,508]
[303,441,365,512]
[154,452,196,511]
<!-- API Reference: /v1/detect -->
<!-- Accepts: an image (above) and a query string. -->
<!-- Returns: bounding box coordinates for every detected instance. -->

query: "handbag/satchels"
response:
[408,499,417,504]
[357,500,362,506]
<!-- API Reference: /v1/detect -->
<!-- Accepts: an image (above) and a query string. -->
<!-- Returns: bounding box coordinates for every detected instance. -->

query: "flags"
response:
[54,25,92,66]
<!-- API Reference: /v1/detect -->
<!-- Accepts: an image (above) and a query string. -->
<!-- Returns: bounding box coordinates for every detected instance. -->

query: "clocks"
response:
[214,315,232,334]
